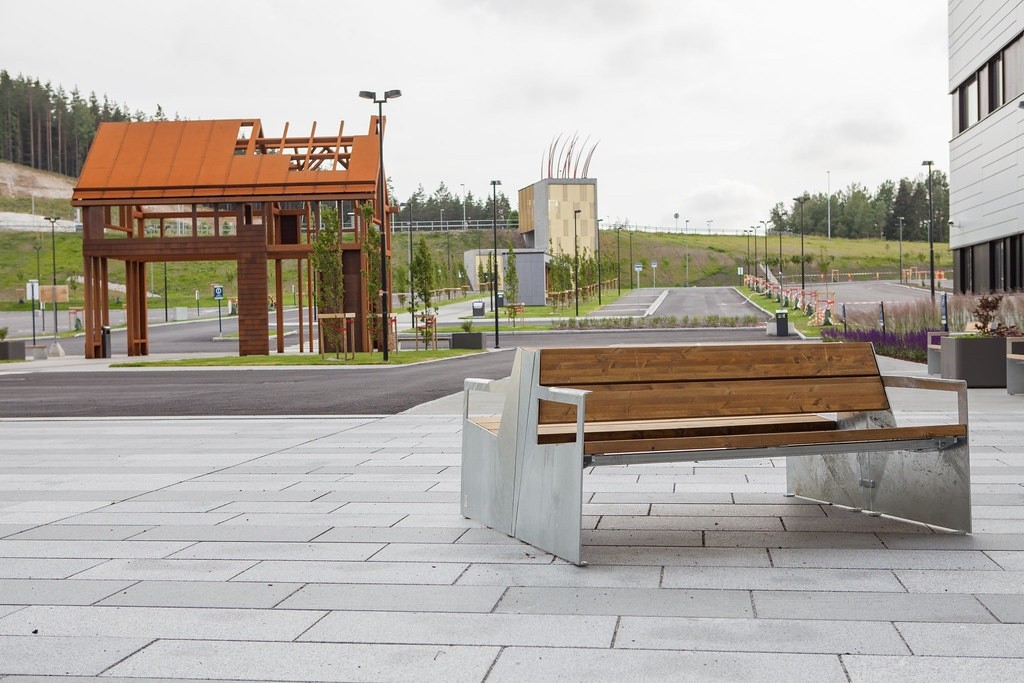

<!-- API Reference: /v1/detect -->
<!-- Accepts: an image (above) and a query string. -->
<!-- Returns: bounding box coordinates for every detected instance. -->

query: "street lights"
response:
[460,182,476,236]
[617,227,622,296]
[574,209,579,317]
[828,169,846,243]
[630,231,636,291]
[440,208,444,230]
[775,212,786,290]
[490,180,508,349]
[743,229,754,276]
[792,196,811,292]
[760,220,772,290]
[920,160,939,307]
[685,219,689,289]
[157,224,171,322]
[358,88,404,363]
[44,214,61,335]
[597,218,605,306]
[897,215,910,286]
[751,226,761,279]
[399,202,414,328]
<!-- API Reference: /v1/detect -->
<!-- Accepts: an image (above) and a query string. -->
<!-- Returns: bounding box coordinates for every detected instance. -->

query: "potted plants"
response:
[939,288,1022,389]
[0,326,26,361]
[452,319,487,350]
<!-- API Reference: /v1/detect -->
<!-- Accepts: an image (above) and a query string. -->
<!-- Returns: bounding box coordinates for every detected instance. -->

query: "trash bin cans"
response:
[498,293,504,306]
[101,325,111,358]
[776,310,788,336]
[472,301,485,316]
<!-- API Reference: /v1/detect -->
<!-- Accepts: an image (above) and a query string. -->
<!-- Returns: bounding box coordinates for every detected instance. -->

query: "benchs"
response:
[458,341,873,536]
[25,345,48,360]
[512,340,973,569]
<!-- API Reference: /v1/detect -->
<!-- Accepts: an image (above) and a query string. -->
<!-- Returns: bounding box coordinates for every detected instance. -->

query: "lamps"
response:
[947,218,961,228]
